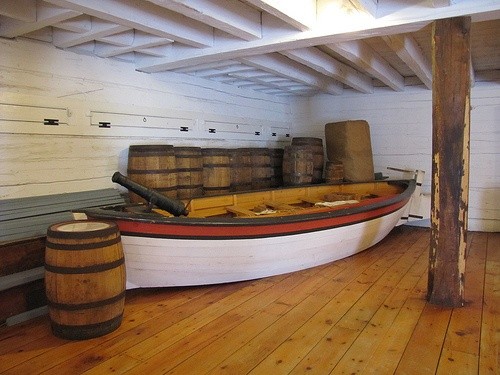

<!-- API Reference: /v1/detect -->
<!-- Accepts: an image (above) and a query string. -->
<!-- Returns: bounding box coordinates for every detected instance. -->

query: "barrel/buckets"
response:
[251,148,271,190]
[325,161,344,183]
[127,145,177,206]
[230,148,251,189]
[271,149,283,188]
[203,148,233,197]
[290,137,324,184]
[44,220,126,338]
[283,146,313,184]
[174,147,204,201]
[325,120,374,183]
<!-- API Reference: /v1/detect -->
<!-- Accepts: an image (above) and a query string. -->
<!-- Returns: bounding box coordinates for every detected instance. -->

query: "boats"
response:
[71,178,417,289]
